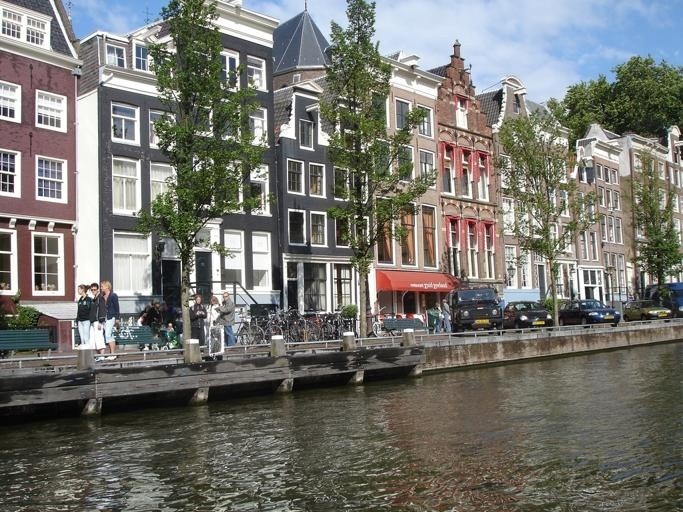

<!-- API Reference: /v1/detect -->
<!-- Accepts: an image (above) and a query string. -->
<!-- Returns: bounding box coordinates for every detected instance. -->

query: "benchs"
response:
[0,329,57,359]
[73,326,161,351]
[380,318,429,336]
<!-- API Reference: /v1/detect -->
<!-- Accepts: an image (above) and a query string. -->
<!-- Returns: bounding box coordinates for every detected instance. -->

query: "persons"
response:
[426,302,444,336]
[76,280,236,362]
[441,299,452,335]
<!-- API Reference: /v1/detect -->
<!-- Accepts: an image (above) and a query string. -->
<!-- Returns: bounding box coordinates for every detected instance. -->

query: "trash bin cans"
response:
[428,307,440,333]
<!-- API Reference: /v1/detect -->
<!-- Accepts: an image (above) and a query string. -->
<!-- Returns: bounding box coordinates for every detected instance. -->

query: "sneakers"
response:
[96,352,118,362]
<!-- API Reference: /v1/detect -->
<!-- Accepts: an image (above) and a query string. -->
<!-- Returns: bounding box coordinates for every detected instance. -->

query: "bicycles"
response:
[227,304,400,349]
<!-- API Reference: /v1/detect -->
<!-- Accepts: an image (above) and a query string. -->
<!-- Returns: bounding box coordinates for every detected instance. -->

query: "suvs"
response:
[450,286,505,337]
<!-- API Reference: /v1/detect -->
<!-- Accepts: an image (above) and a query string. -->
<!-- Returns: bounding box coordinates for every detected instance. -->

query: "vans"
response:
[644,282,683,318]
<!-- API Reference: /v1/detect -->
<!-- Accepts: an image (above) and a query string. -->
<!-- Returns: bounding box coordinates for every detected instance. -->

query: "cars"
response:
[500,299,554,332]
[622,300,673,323]
[559,298,622,329]
